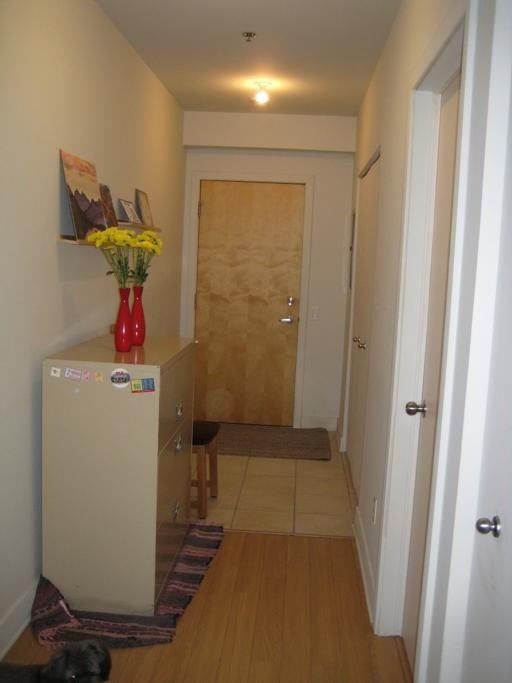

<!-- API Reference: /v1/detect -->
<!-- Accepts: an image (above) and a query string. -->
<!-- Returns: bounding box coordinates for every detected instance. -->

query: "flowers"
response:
[86,222,165,286]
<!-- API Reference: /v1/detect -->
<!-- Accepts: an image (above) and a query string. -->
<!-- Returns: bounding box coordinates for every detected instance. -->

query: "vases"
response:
[132,286,147,346]
[114,286,133,351]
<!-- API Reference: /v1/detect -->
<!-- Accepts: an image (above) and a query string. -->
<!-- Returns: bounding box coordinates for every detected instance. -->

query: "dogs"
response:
[0,641,113,683]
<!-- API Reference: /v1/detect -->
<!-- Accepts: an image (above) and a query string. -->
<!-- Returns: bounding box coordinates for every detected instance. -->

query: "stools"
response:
[190,418,221,518]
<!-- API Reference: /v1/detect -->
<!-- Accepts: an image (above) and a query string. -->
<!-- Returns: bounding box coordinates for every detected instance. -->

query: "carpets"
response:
[191,421,334,461]
[31,518,228,653]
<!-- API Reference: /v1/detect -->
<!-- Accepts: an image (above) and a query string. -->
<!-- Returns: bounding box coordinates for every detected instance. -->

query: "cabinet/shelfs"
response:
[40,333,201,619]
